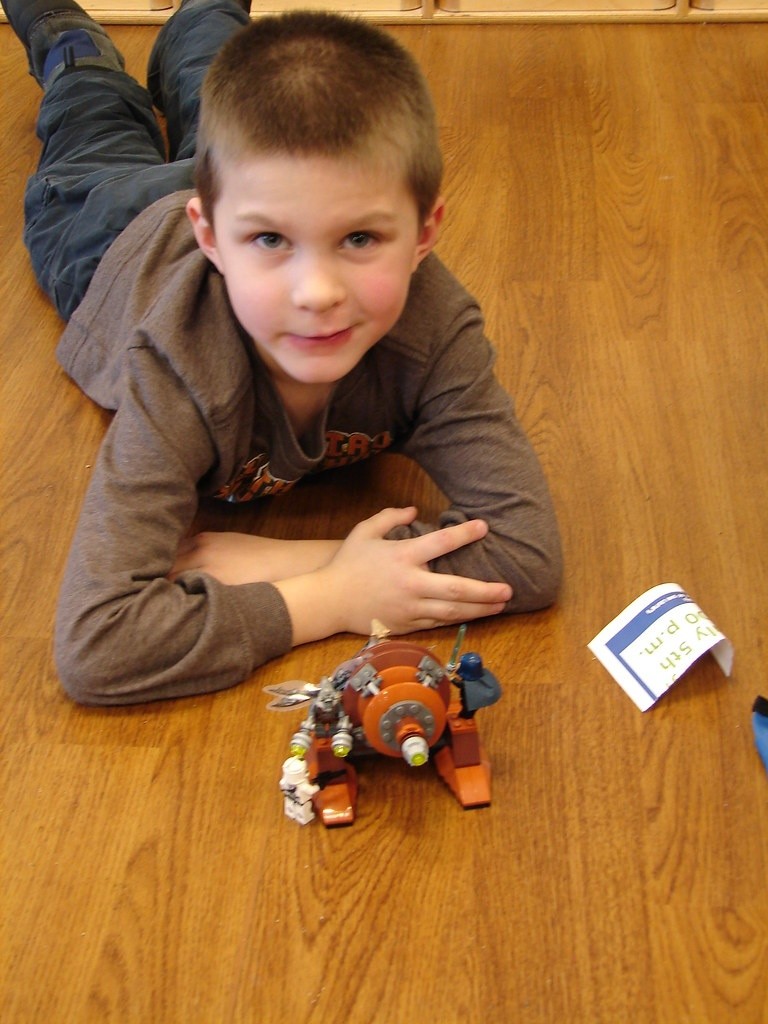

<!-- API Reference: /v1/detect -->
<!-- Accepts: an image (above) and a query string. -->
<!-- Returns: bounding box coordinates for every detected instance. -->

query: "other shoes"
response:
[180,0,251,15]
[1,0,126,91]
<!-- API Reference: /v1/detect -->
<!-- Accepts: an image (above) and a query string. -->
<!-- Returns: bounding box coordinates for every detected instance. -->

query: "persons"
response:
[3,0,561,709]
